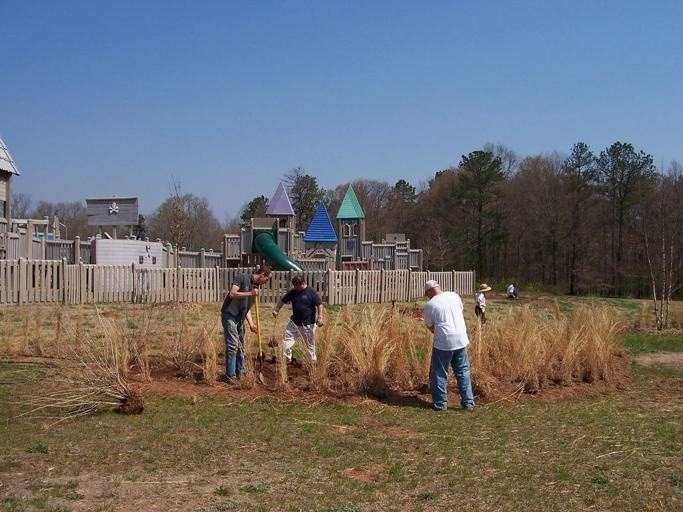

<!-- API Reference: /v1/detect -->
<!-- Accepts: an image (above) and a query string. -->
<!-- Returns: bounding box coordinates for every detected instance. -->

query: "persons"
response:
[221,264,273,383]
[423,279,475,412]
[137,229,148,241]
[273,277,325,365]
[474,283,492,323]
[506,281,518,300]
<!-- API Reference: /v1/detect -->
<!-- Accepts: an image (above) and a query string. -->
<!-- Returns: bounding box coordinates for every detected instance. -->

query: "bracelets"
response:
[318,315,323,318]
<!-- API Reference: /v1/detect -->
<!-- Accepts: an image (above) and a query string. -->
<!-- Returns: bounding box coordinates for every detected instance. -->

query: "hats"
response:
[423,278,440,296]
[476,283,492,293]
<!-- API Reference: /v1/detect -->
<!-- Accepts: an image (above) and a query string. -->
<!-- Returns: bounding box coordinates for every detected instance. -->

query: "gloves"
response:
[315,316,324,327]
[272,309,281,318]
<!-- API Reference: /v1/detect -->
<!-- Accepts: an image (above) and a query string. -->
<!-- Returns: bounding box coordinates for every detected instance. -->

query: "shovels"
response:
[422,358,432,394]
[255,296,270,386]
